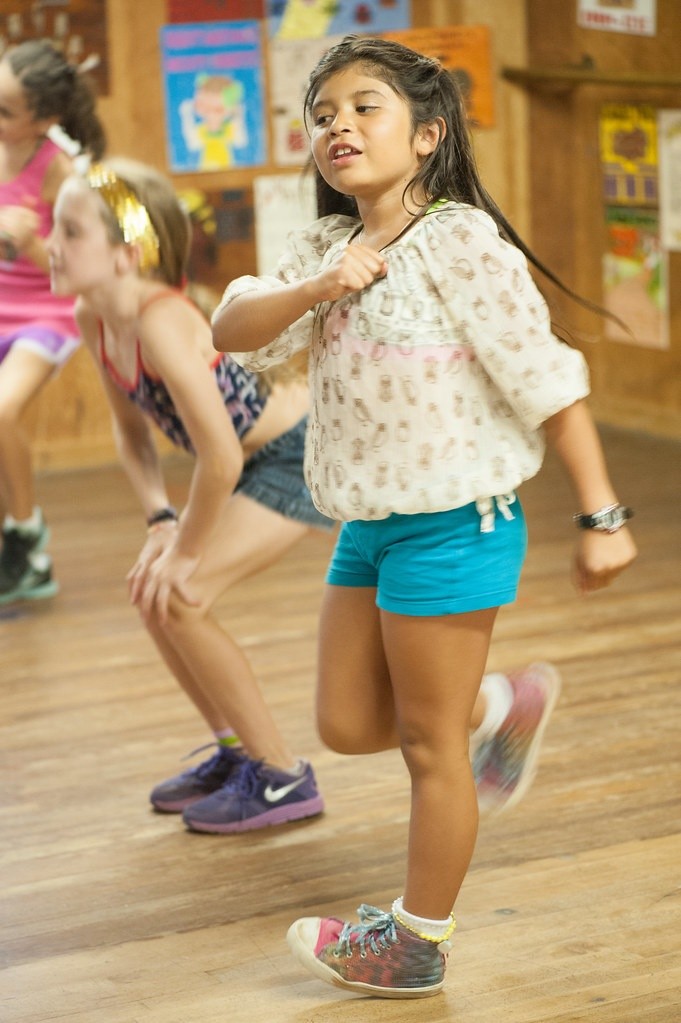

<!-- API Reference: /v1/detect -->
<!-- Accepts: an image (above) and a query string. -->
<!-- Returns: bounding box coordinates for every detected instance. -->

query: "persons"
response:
[49,162,343,835]
[215,33,640,997]
[0,40,107,596]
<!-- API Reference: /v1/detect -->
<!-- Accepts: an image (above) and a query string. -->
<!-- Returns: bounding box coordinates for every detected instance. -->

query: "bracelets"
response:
[146,509,177,532]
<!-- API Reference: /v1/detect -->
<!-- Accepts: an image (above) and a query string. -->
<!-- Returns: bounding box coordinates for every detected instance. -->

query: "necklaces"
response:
[309,196,438,349]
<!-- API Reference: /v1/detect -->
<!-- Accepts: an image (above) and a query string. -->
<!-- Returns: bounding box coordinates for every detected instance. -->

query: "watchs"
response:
[575,503,629,533]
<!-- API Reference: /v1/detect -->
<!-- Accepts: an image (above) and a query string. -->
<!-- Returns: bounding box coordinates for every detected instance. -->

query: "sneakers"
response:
[1,506,50,552]
[181,759,324,832]
[1,552,58,605]
[468,661,563,819]
[285,914,447,1000]
[150,747,249,811]
